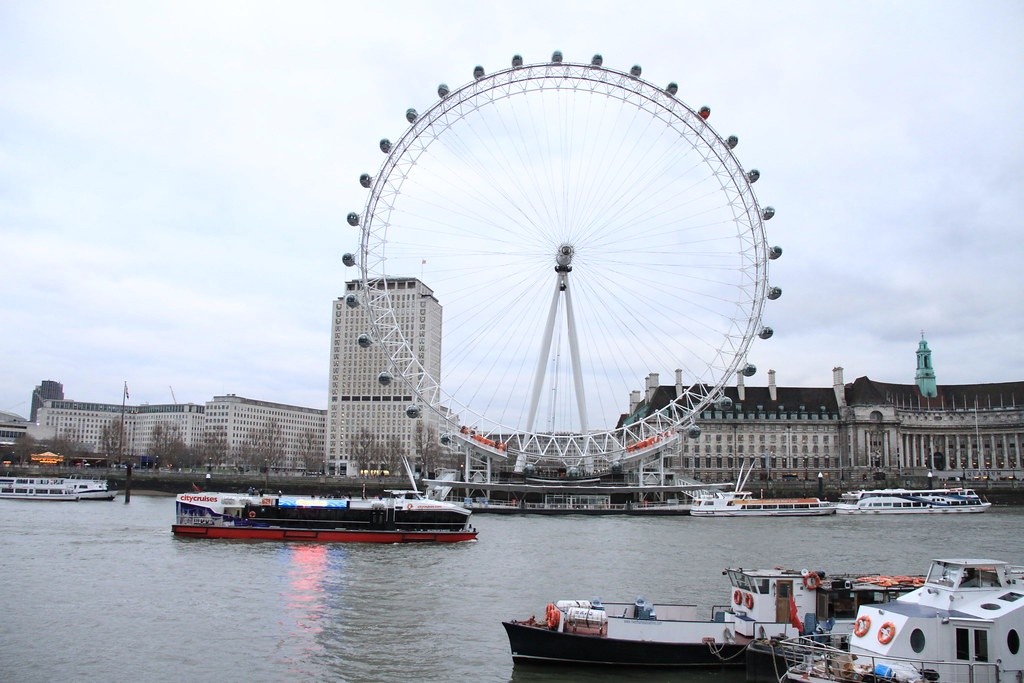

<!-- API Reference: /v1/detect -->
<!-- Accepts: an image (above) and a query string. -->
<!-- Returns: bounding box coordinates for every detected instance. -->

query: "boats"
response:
[836,486,992,515]
[689,457,836,516]
[498,557,1024,682]
[169,473,479,545]
[0,470,119,502]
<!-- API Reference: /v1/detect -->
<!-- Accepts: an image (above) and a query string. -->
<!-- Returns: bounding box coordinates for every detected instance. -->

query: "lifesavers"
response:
[857,574,925,586]
[744,594,753,608]
[249,511,258,518]
[877,621,895,644]
[734,590,743,605]
[803,573,821,590]
[545,603,561,629]
[854,616,872,637]
